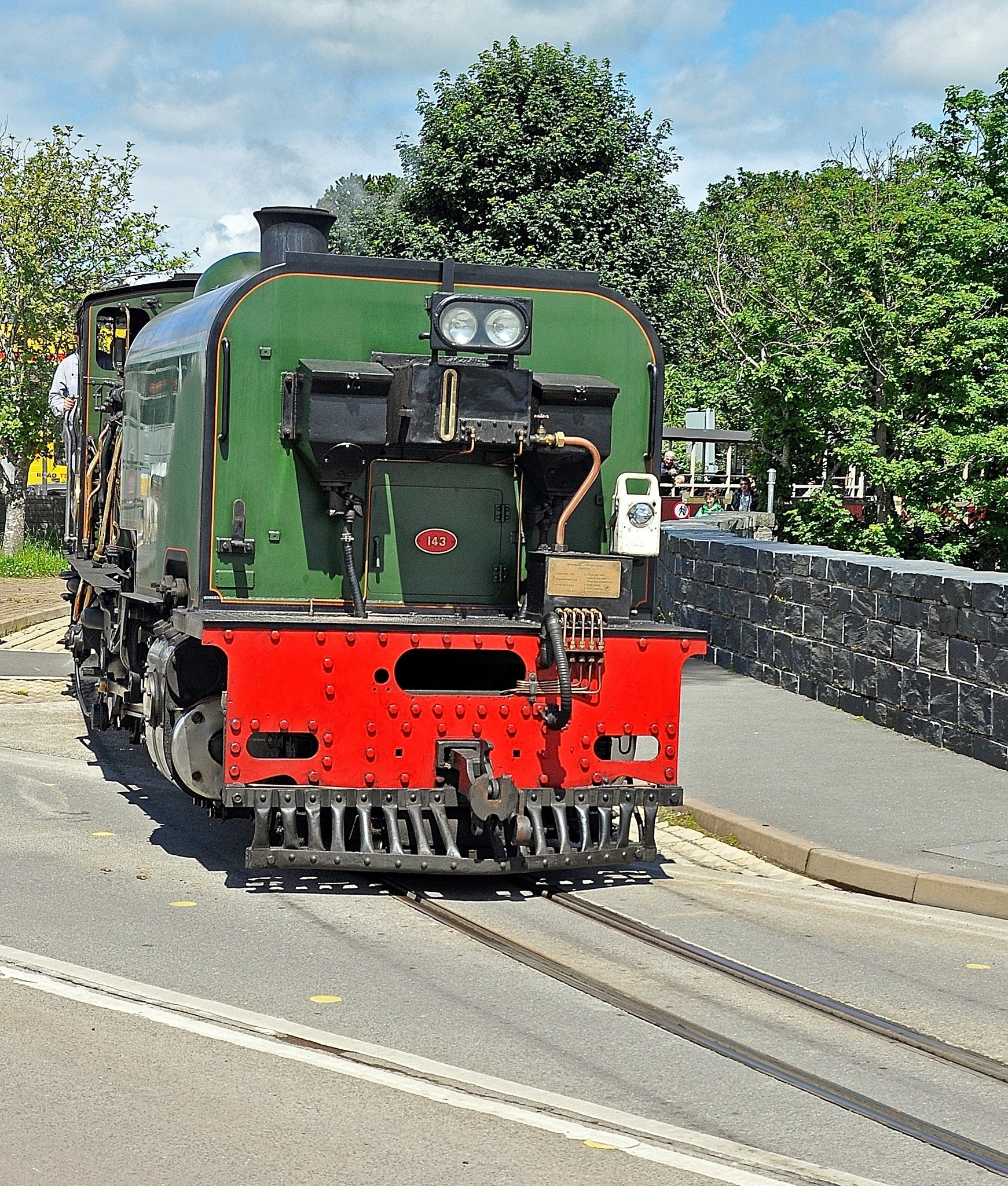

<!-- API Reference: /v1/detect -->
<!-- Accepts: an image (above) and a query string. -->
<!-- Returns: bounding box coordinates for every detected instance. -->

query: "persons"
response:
[695,491,724,518]
[48,334,113,520]
[660,450,728,497]
[730,477,759,512]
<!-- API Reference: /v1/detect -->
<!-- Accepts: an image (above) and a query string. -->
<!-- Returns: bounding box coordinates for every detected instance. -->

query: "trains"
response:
[60,202,713,889]
